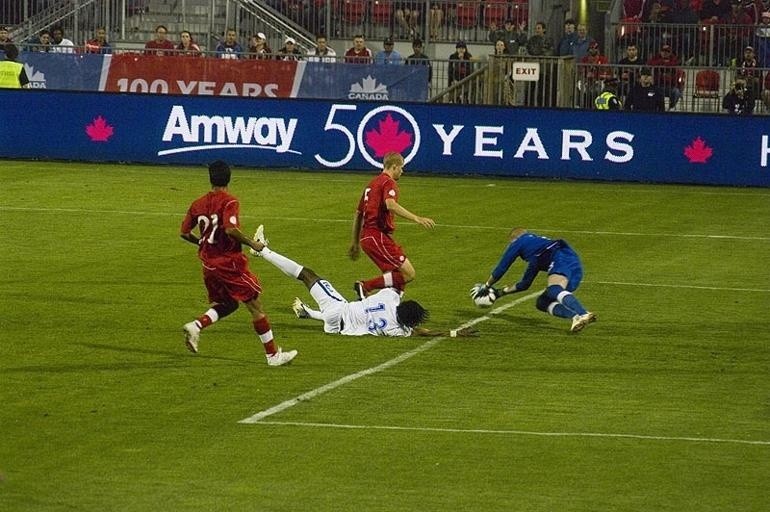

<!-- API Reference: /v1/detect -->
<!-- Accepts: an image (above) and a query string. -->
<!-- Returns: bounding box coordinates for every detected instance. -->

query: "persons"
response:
[250,224,480,338]
[349,151,435,300]
[180,160,298,366]
[470,226,598,333]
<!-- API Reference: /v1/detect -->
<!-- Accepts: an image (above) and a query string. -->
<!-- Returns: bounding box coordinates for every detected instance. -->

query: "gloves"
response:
[496,286,508,298]
[470,283,489,300]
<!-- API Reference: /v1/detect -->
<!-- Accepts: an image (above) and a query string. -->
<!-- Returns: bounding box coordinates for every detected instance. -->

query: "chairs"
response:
[268,1,720,113]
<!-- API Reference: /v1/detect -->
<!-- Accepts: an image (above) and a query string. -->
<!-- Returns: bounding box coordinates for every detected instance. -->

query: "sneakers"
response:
[291,297,308,320]
[183,321,200,354]
[265,349,298,367]
[354,280,367,302]
[571,312,596,333]
[250,224,269,257]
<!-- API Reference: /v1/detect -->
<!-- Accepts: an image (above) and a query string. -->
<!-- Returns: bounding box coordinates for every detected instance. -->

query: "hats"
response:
[383,38,394,45]
[588,41,599,49]
[253,32,267,40]
[744,45,753,51]
[761,11,770,18]
[285,38,296,44]
[661,45,672,52]
[455,41,467,48]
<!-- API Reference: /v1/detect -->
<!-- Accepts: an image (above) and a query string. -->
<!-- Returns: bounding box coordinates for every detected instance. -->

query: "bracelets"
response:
[449,330,457,338]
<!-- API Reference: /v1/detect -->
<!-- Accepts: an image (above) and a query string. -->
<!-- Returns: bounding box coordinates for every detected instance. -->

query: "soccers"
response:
[475,286,496,306]
